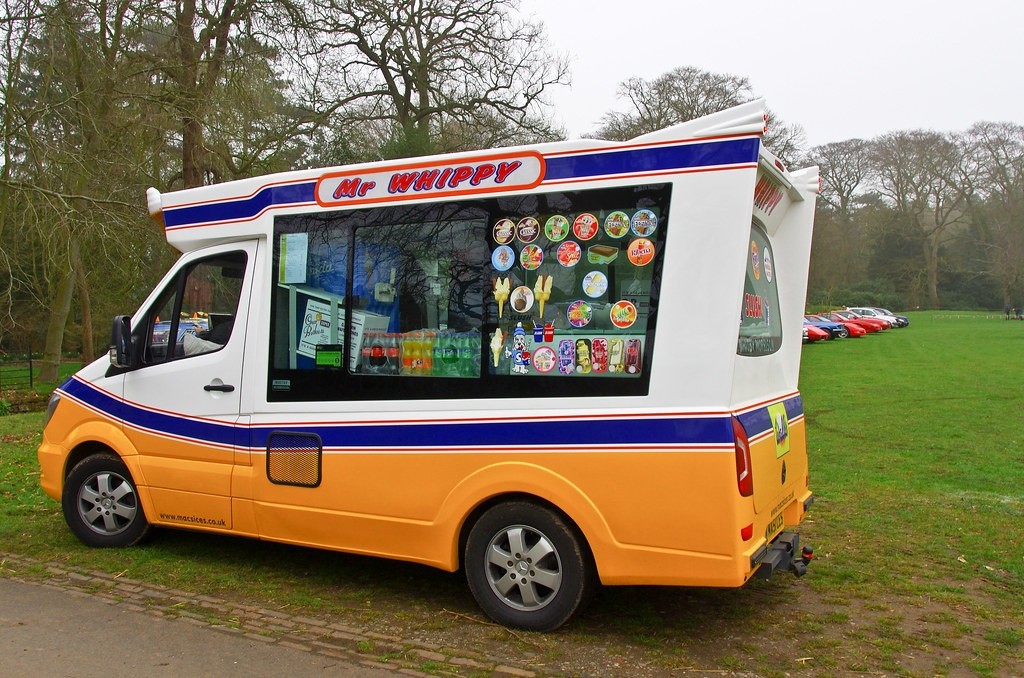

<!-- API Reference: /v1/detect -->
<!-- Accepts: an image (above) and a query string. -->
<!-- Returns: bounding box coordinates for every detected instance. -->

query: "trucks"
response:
[37,97,823,633]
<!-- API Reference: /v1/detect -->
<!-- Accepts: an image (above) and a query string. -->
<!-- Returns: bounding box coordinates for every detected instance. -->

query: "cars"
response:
[805,315,845,340]
[848,311,891,329]
[818,312,866,338]
[875,308,909,326]
[846,307,904,329]
[838,311,882,332]
[802,318,829,343]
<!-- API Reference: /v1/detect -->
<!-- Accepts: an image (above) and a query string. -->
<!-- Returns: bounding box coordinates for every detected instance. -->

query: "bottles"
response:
[361,329,481,378]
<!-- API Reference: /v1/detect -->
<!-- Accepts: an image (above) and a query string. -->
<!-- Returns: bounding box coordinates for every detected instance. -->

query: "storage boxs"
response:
[299,299,390,371]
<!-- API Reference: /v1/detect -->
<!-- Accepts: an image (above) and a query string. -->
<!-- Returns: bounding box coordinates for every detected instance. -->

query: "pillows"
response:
[183,333,224,357]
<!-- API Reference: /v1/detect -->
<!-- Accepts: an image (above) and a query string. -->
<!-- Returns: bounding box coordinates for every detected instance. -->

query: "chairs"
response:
[209,320,234,344]
